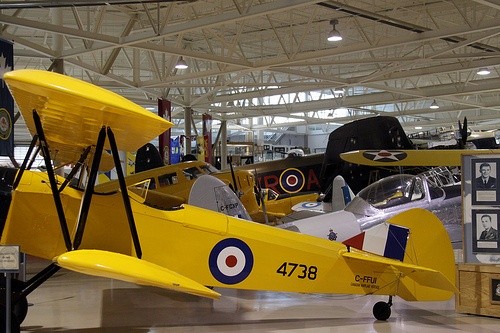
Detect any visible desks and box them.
[455,263,500,318]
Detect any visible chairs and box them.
[387,177,416,207]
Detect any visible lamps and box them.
[175,56,188,69]
[327,19,342,42]
[430,99,439,109]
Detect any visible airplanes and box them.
[0,60,500,333]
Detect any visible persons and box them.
[181,154,218,180]
[479,215,498,240]
[475,164,497,189]
